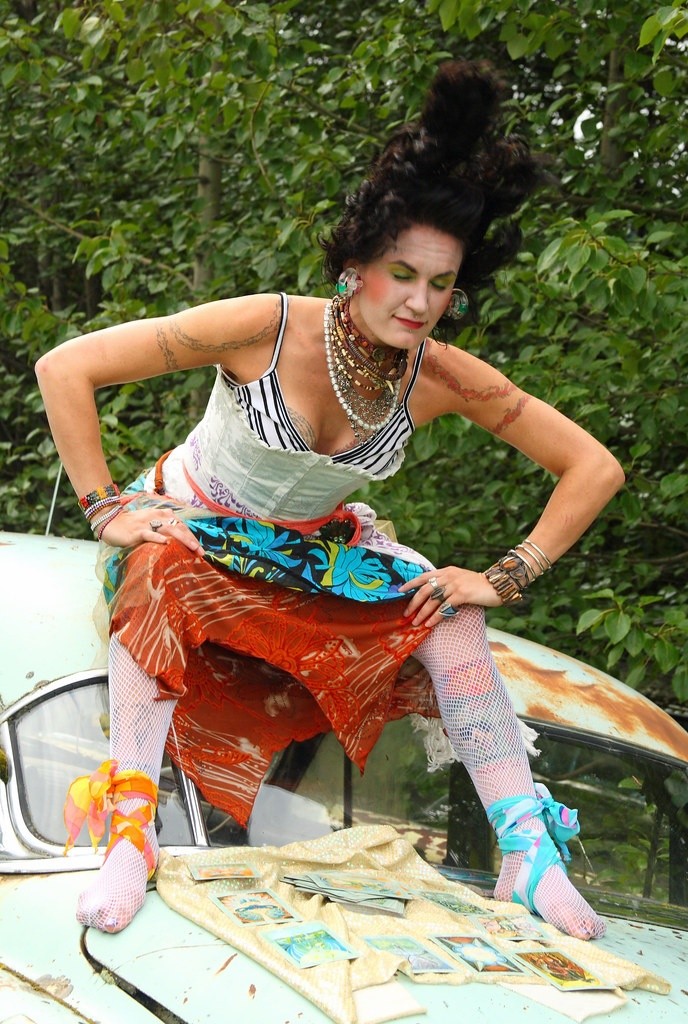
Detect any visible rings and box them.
[439,603,459,618]
[431,586,447,602]
[149,520,162,532]
[169,518,179,526]
[429,577,437,589]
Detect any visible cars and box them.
[1,531,688,1024]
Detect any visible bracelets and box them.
[77,483,124,540]
[485,540,552,607]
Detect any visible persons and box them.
[35,62,626,943]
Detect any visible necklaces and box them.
[324,293,409,446]
